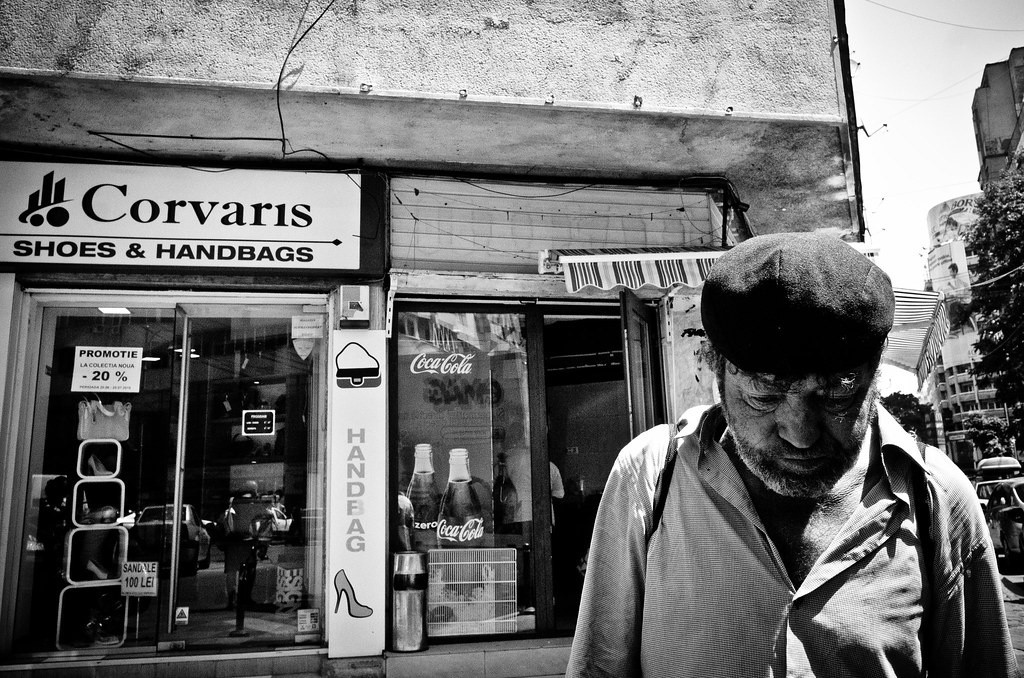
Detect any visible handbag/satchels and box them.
[494,452,518,529]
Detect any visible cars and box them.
[137,503,210,570]
[973,476,1024,553]
[974,457,1022,475]
[224,502,292,539]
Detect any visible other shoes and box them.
[950,328,962,333]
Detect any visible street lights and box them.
[948,263,981,411]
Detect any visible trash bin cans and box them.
[391,550,428,653]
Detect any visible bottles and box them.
[494,451,519,534]
[401,443,484,549]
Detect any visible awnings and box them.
[878,286,949,392]
[543,244,732,293]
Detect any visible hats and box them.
[700,233,895,374]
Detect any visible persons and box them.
[948,263,969,292]
[565,233,1020,678]
[507,446,564,612]
